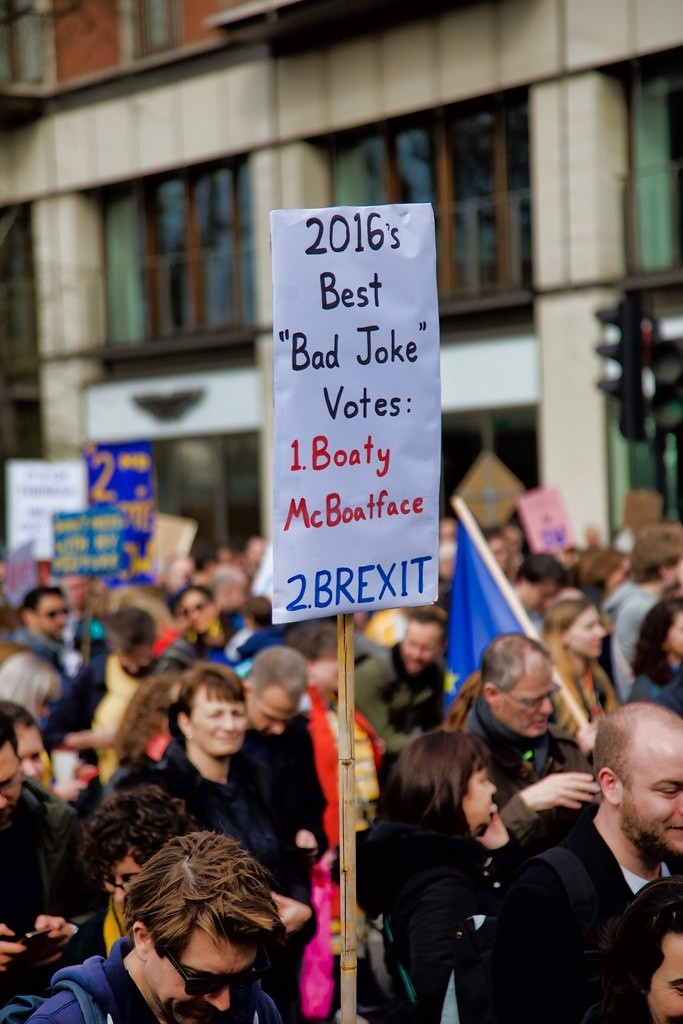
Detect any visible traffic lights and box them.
[594,290,647,444]
[647,336,683,436]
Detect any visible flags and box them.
[441,519,532,716]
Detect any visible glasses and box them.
[495,682,560,709]
[47,607,70,618]
[165,946,271,996]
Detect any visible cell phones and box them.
[21,927,53,946]
[475,812,494,836]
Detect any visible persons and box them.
[0,518,682,1024]
